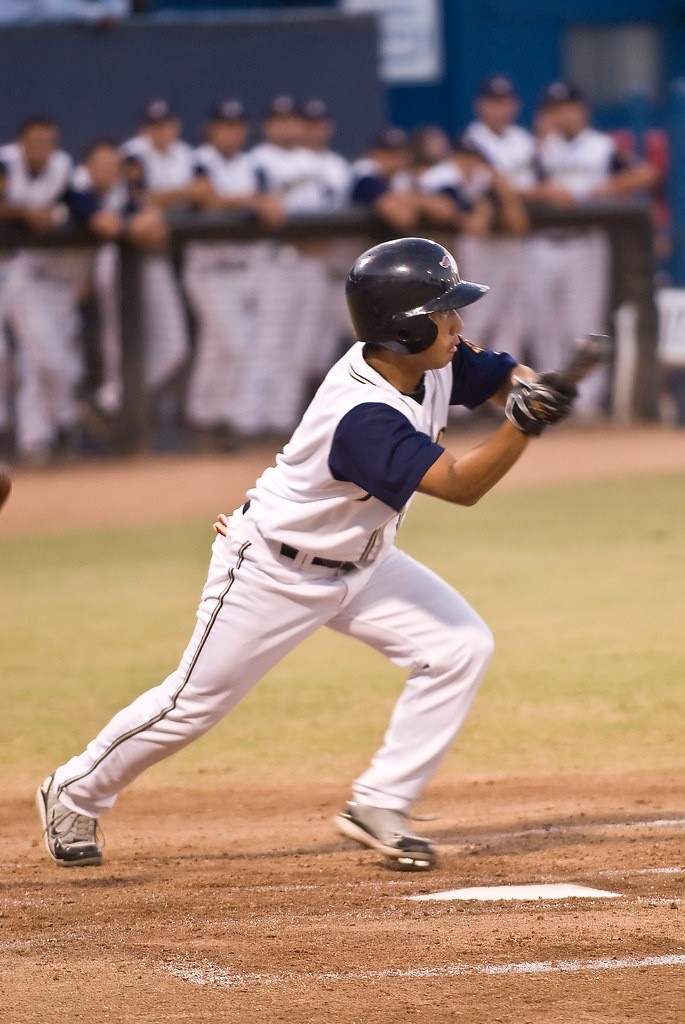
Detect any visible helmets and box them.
[345,237,491,352]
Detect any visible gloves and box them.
[505,371,577,434]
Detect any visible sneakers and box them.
[35,773,106,867]
[335,807,435,867]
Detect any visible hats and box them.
[303,100,328,119]
[475,76,517,99]
[142,99,177,123]
[209,99,246,122]
[371,127,410,151]
[263,95,299,119]
[537,83,581,105]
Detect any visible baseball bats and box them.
[553,331,612,392]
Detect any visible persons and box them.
[35,236,581,869]
[0,79,685,457]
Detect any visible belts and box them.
[243,500,357,571]
